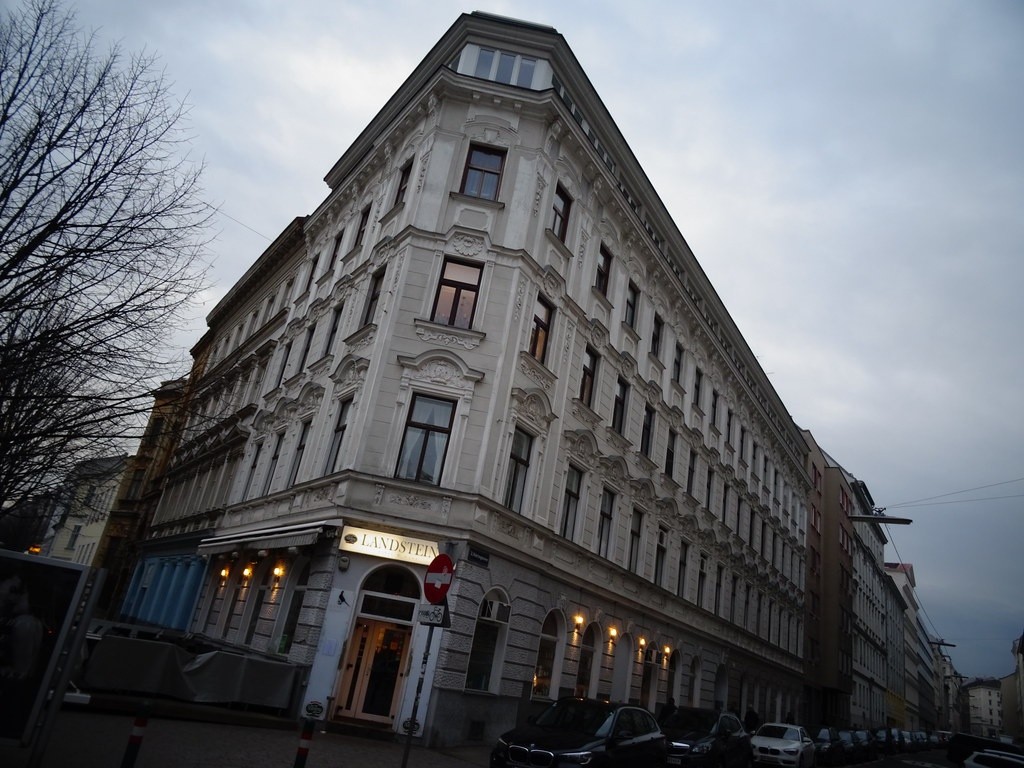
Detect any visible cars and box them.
[947,732,1024,763]
[749,722,817,768]
[806,725,952,768]
[488,694,667,768]
[662,705,751,768]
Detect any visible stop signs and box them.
[423,553,454,604]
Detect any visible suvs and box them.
[959,748,1024,768]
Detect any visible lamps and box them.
[243,568,251,582]
[272,567,281,584]
[664,646,670,658]
[218,569,226,584]
[639,639,646,649]
[610,629,617,640]
[575,616,584,630]
